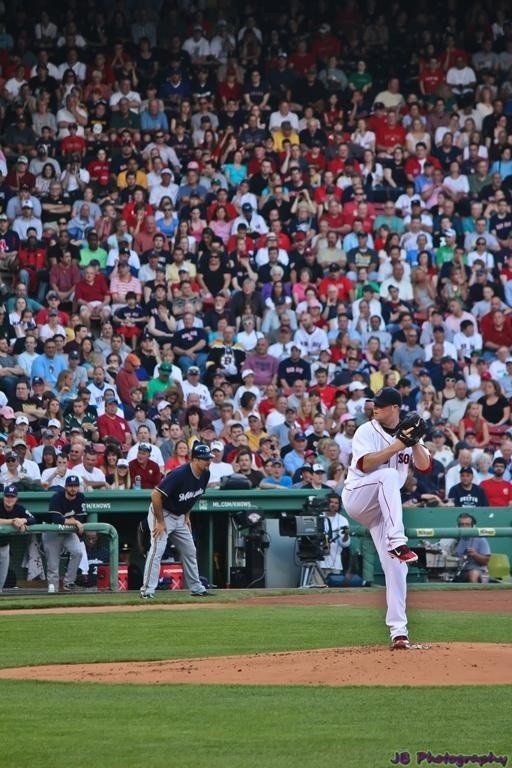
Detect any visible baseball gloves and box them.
[395,411,426,446]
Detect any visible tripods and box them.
[297,562,328,589]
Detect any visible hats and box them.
[112,243,204,287]
[387,281,398,294]
[262,231,283,253]
[355,229,370,240]
[239,195,261,219]
[154,161,184,189]
[65,476,79,486]
[4,486,17,497]
[173,66,253,86]
[190,174,222,207]
[324,259,346,281]
[0,376,409,481]
[3,140,48,236]
[374,98,390,115]
[471,266,490,281]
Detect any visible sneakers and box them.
[191,591,214,596]
[385,545,418,564]
[140,594,156,599]
[390,636,410,648]
[63,583,87,592]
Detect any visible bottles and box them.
[135,474,142,490]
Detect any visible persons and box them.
[340,387,433,650]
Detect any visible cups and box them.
[48,583,56,593]
